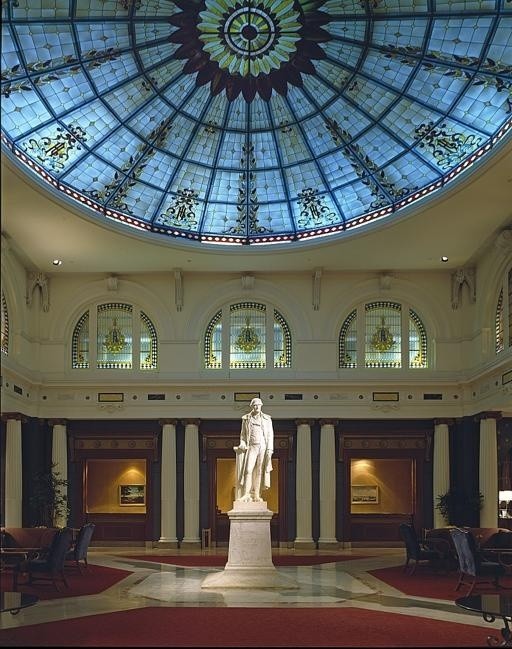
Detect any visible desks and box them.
[456,595,512,648]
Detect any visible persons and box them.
[237,397,274,501]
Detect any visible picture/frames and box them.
[118,484,146,506]
[351,484,378,504]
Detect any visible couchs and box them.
[428,528,512,570]
[0,527,59,568]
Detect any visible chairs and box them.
[13,523,96,592]
[400,523,441,578]
[449,527,504,598]
